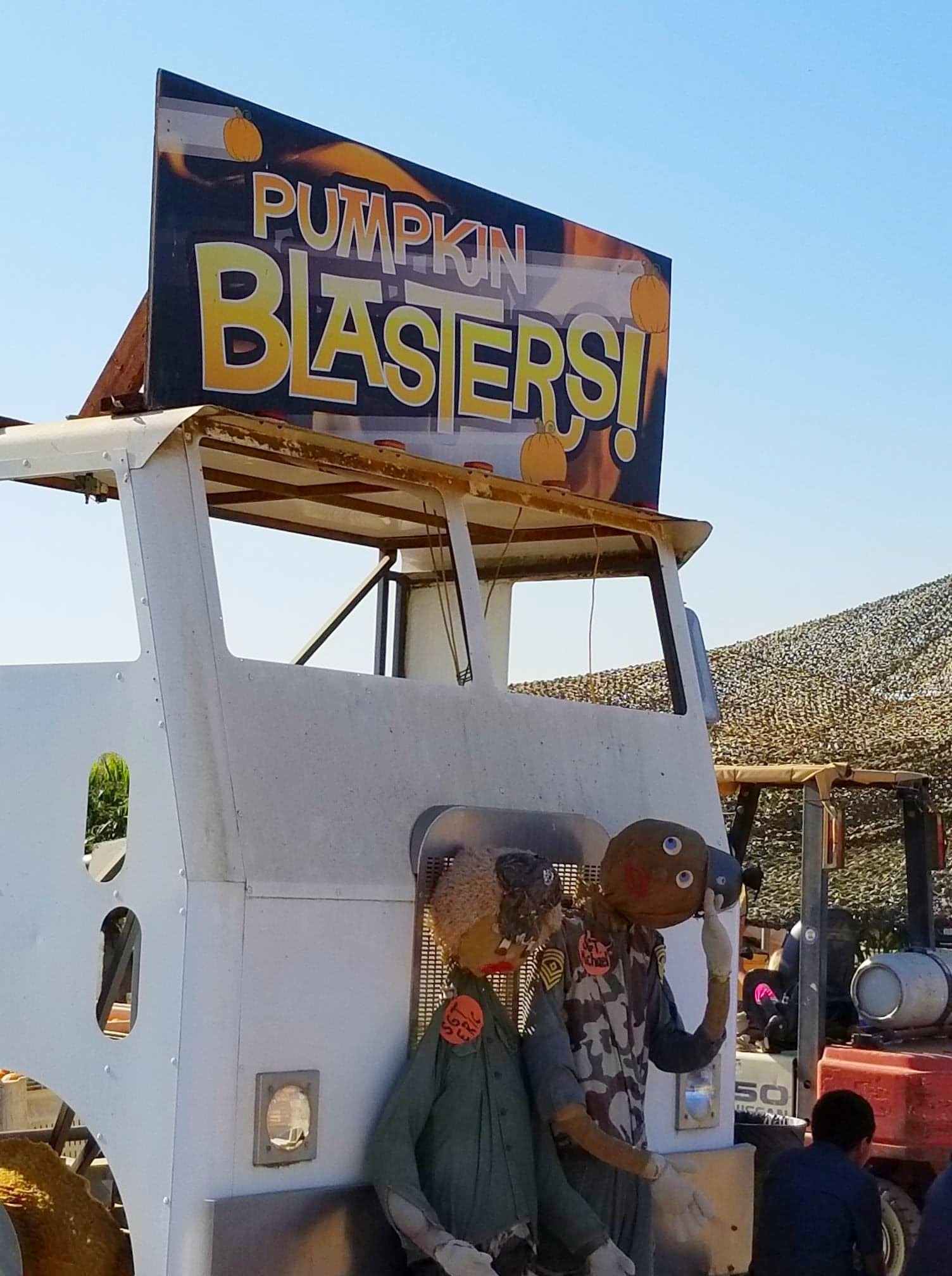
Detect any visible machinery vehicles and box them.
[712,760,951,1275]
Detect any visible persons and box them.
[906,1164,952,1275]
[751,1089,887,1276]
